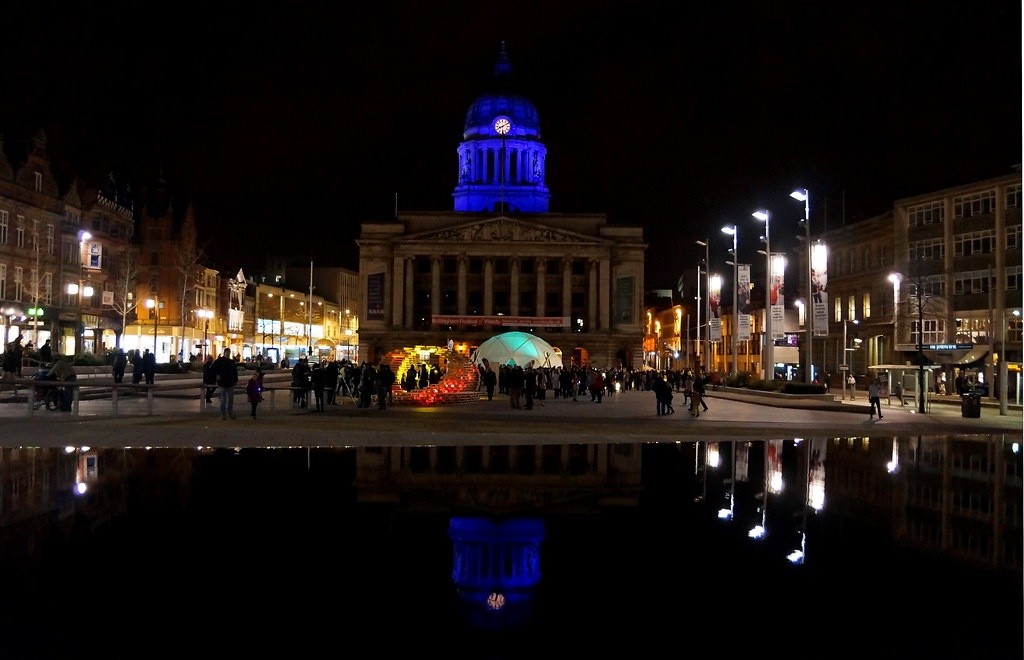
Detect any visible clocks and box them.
[494,116,512,135]
[487,592,505,610]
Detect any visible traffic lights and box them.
[28,305,59,320]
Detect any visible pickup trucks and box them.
[0,358,48,377]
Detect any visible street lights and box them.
[64,229,93,355]
[748,439,770,538]
[887,272,927,415]
[346,330,352,361]
[789,189,812,385]
[199,309,214,361]
[840,319,861,400]
[674,352,679,371]
[696,238,709,374]
[752,209,774,380]
[786,438,812,565]
[722,223,738,380]
[717,441,738,521]
[999,311,1020,415]
[146,276,165,360]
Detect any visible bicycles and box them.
[29,390,62,412]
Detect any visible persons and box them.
[1,334,201,414]
[202,351,292,371]
[813,371,834,392]
[290,355,445,412]
[245,372,263,419]
[709,279,785,319]
[214,347,240,422]
[476,357,731,418]
[936,371,982,400]
[894,381,904,404]
[811,268,825,302]
[203,355,219,404]
[867,379,883,420]
[254,365,266,401]
[847,373,858,400]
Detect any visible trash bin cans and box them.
[961,393,980,418]
